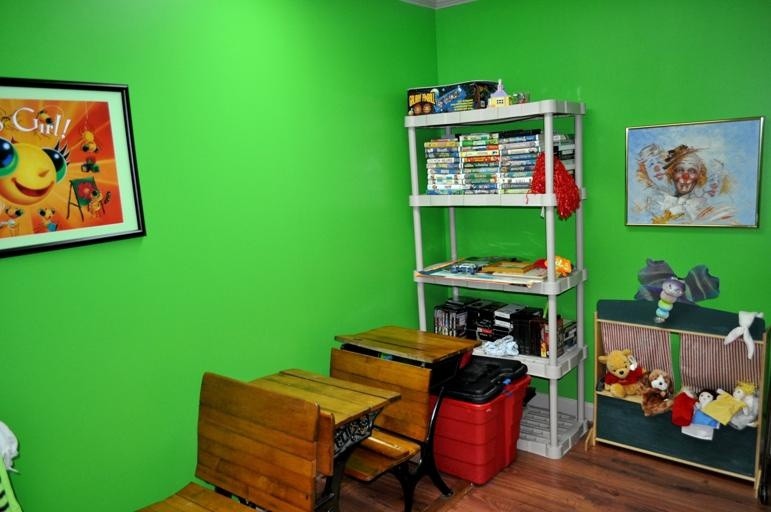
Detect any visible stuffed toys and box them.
[693,389,722,431]
[670,386,698,427]
[598,348,652,399]
[701,380,756,425]
[652,277,685,324]
[635,367,675,418]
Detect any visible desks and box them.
[248,367,403,512]
[335,325,482,368]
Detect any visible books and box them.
[433,296,577,358]
[413,256,546,287]
[414,130,576,196]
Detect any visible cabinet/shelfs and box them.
[591,310,771,503]
[403,100,589,459]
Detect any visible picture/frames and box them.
[0,77,147,258]
[624,115,765,228]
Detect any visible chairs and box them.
[329,348,454,512]
[133,372,335,512]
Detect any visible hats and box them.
[662,145,700,170]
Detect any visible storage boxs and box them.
[429,354,531,486]
